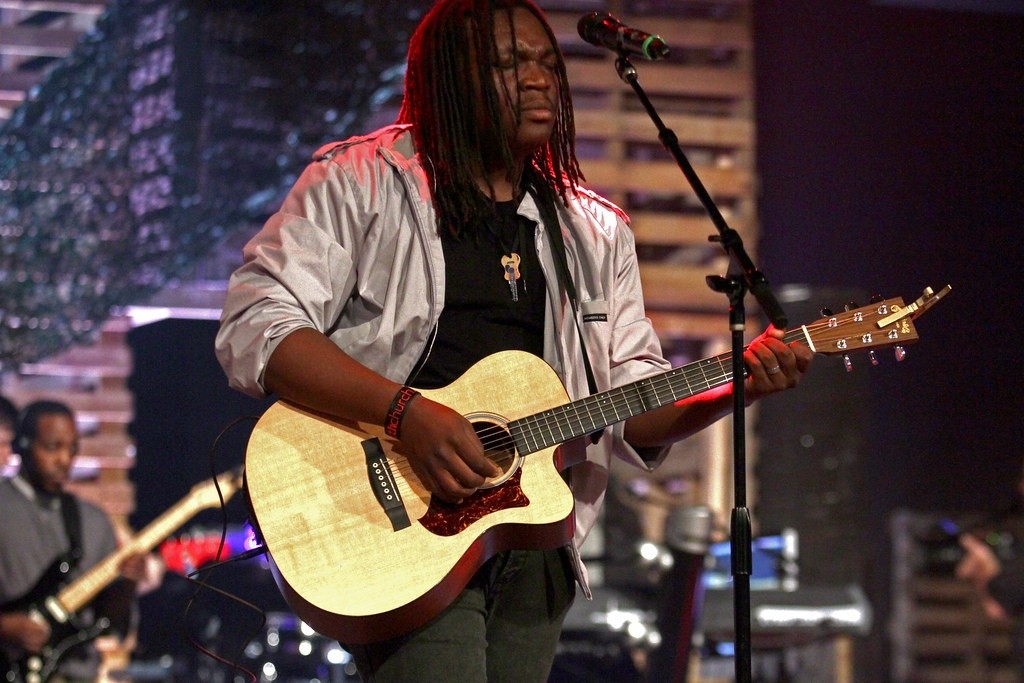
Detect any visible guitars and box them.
[1,460,246,683]
[240,282,956,649]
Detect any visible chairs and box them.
[892,511,1024,683]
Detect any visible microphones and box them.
[578,11,671,60]
[650,503,711,683]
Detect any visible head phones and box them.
[12,401,43,453]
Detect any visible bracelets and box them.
[386,385,420,438]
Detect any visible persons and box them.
[0,399,148,683]
[956,531,1023,620]
[215,0,812,682]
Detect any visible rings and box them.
[767,366,781,375]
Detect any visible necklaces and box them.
[485,200,521,302]
